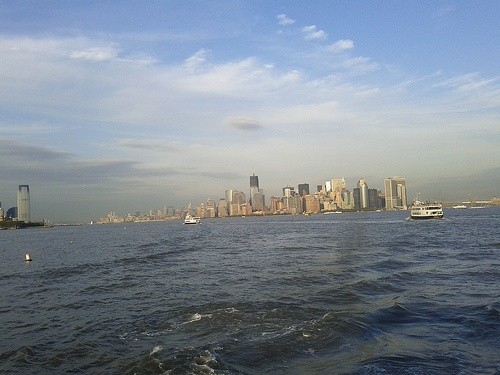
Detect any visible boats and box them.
[470,201,492,210]
[410,205,444,220]
[184,214,201,225]
[323,211,342,215]
[452,202,471,209]
